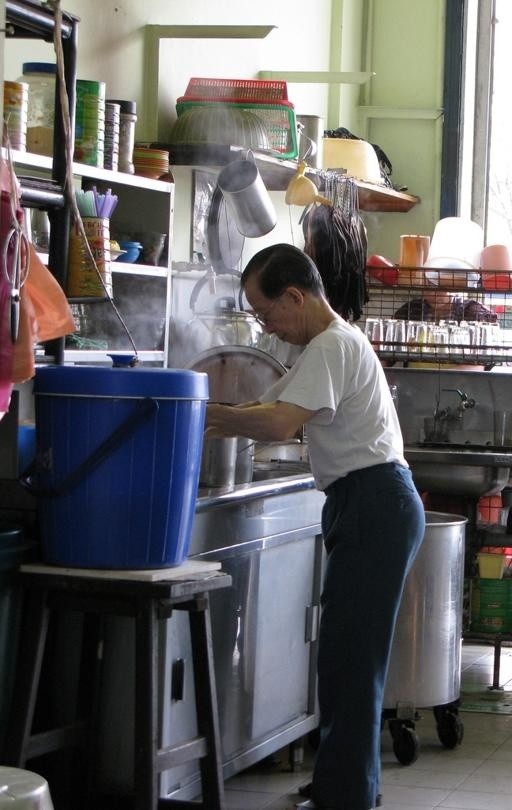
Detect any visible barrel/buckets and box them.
[35,355,209,569]
[423,217,484,286]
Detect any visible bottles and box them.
[35,212,51,248]
[109,98,139,175]
[18,59,67,157]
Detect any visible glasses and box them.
[256,285,291,327]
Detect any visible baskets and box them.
[173,76,299,161]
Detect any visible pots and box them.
[203,426,300,489]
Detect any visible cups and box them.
[216,158,277,237]
[365,317,502,358]
[494,410,512,445]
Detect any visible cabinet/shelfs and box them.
[0,147,175,368]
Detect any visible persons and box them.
[200,241,426,809]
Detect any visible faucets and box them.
[459,399,476,418]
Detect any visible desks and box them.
[12,573,232,810]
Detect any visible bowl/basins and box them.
[120,242,143,263]
[321,137,381,185]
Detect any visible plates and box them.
[110,249,128,260]
[134,145,171,180]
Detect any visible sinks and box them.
[403,446,512,498]
